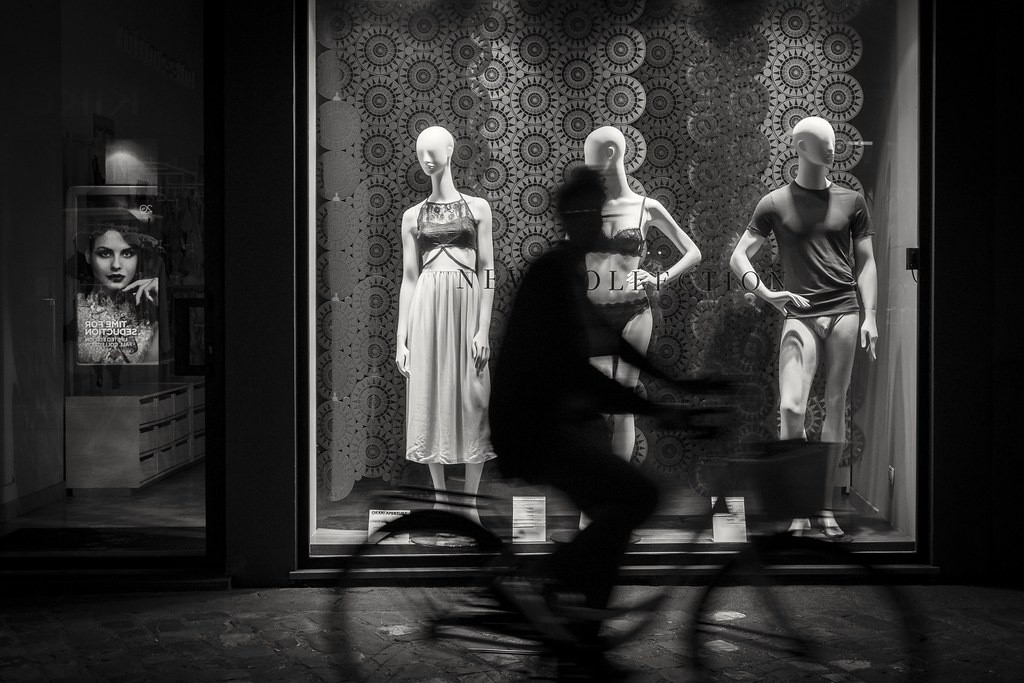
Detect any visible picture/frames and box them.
[63,185,178,396]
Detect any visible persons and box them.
[395,125,498,529]
[730,117,879,540]
[71,215,159,364]
[488,170,746,680]
[578,125,703,529]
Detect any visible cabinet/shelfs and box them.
[125,374,205,468]
[65,382,192,489]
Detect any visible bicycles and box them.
[327,370,935,683]
